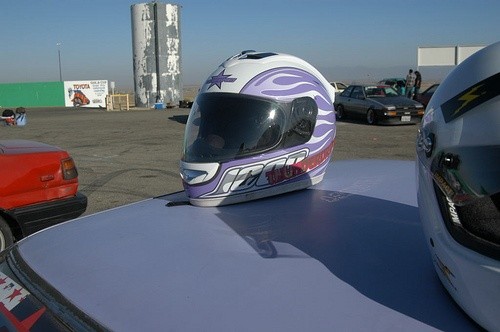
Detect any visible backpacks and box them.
[2,110,15,119]
[16,108,25,113]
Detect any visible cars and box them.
[330,82,347,92]
[415,83,441,108]
[335,83,426,126]
[0,138,88,252]
[0,157,492,332]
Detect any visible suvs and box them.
[375,78,415,95]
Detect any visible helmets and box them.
[416,41,500,332]
[180,50,335,208]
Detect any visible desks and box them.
[106,93,129,111]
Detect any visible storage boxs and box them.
[155,103,163,109]
[0,115,14,126]
[16,113,26,125]
